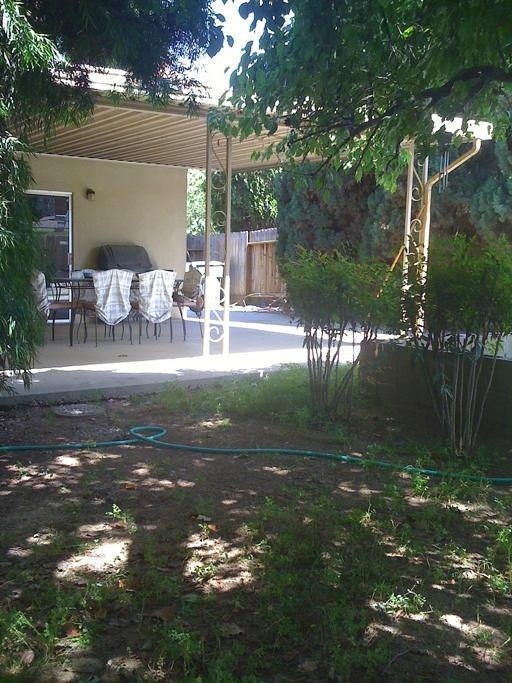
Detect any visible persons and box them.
[30,268,51,322]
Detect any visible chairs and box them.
[43,267,208,348]
